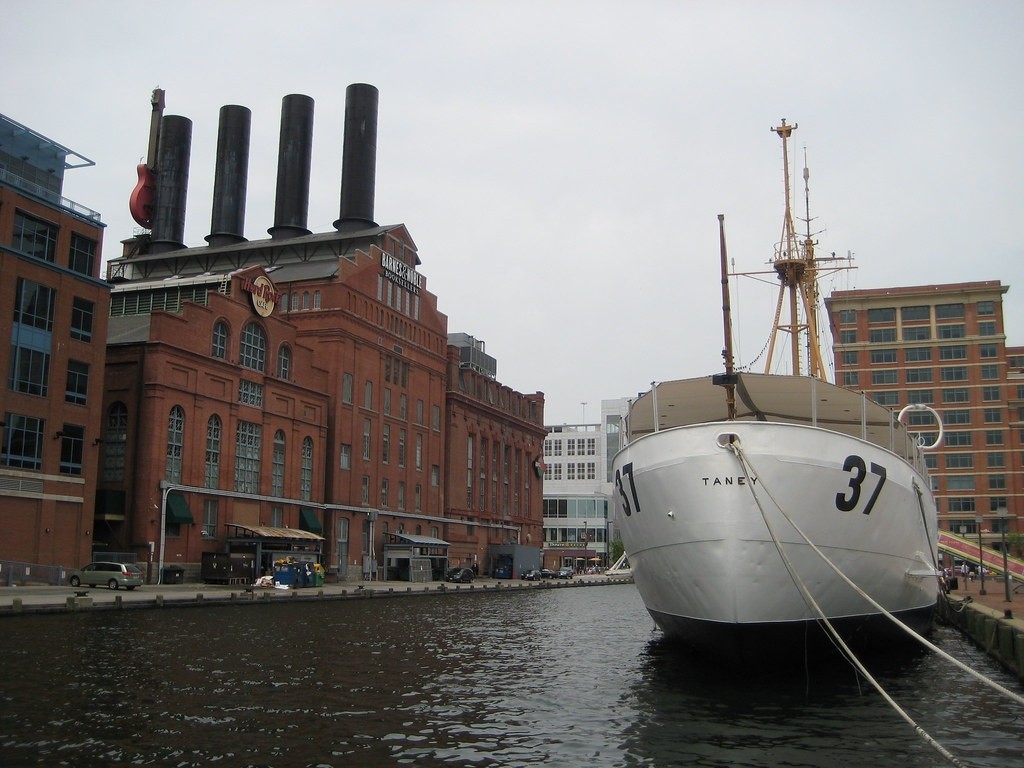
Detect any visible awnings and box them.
[226,523,326,542]
[299,508,322,533]
[383,532,450,549]
[166,492,193,524]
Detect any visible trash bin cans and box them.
[273,560,326,588]
[161,565,185,585]
[950,576,958,590]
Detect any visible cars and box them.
[68,562,143,590]
[521,569,542,581]
[446,567,474,583]
[556,568,573,579]
[945,566,969,576]
[540,570,557,579]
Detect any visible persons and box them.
[576,564,582,574]
[592,565,598,572]
[960,561,975,582]
[939,562,945,577]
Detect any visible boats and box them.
[611,119,940,670]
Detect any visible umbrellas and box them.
[588,558,602,560]
[575,558,585,561]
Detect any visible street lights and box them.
[999,507,1012,602]
[583,520,587,574]
[606,521,613,570]
[960,525,967,590]
[975,515,986,595]
